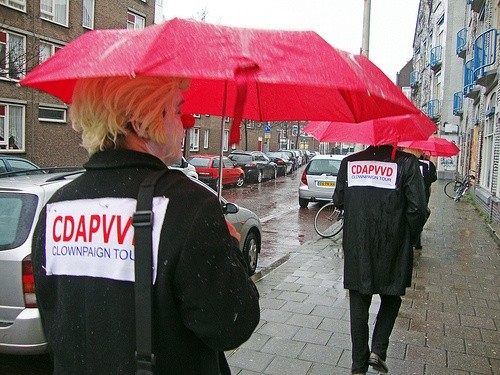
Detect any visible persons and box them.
[30,76,260,375]
[331,143,432,375]
[411,149,438,252]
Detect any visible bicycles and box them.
[443,169,476,203]
[314,200,346,238]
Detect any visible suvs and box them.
[228,149,278,184]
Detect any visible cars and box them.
[341,146,357,155]
[0,165,262,355]
[166,155,198,180]
[265,152,294,176]
[0,154,47,178]
[298,154,350,209]
[185,154,246,192]
[279,148,321,171]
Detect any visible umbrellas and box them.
[14,17,421,209]
[396,137,461,158]
[302,110,439,147]
